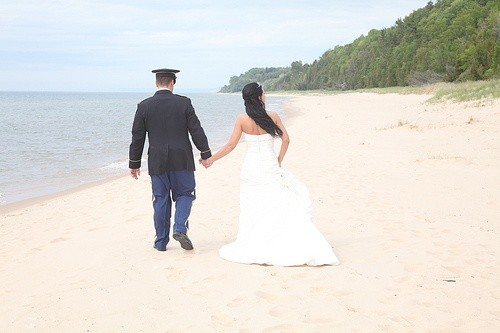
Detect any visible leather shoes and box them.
[173,231,193,251]
[153,244,166,251]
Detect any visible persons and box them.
[128,69,212,252]
[200,82,339,267]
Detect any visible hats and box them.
[151,69,181,79]
